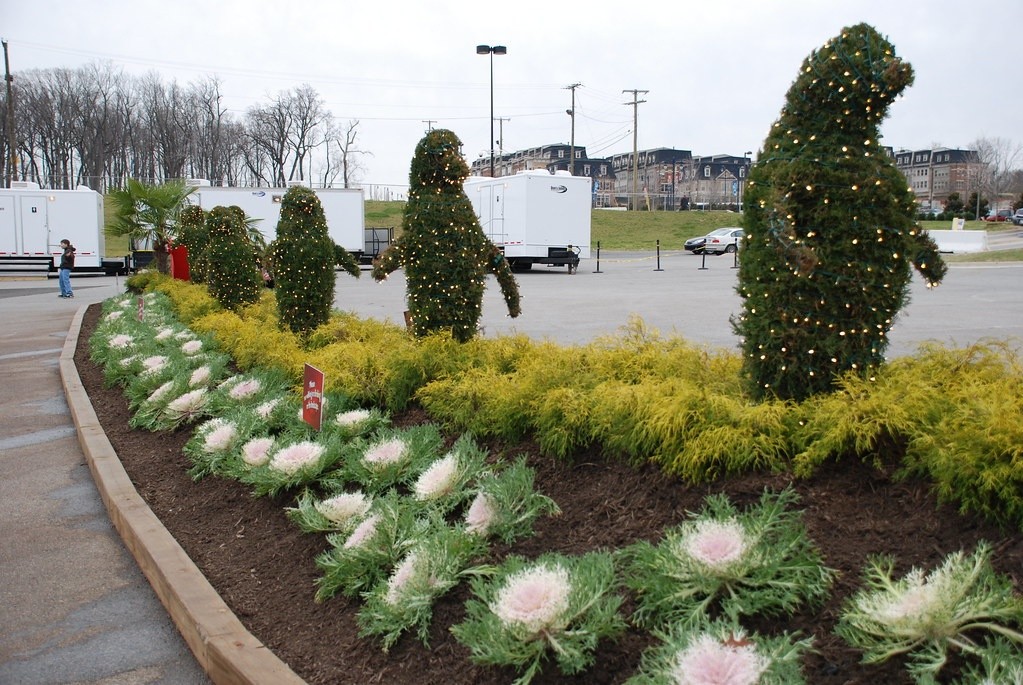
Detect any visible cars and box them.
[1012,208,1023,225]
[704,228,745,253]
[920,199,944,217]
[684,227,727,255]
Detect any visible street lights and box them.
[475,45,508,178]
[744,151,752,189]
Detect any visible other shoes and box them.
[58,295,74,299]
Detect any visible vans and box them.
[987,208,1012,222]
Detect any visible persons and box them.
[58,240,76,298]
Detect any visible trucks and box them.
[129,178,366,255]
[455,167,591,273]
[0,181,106,271]
